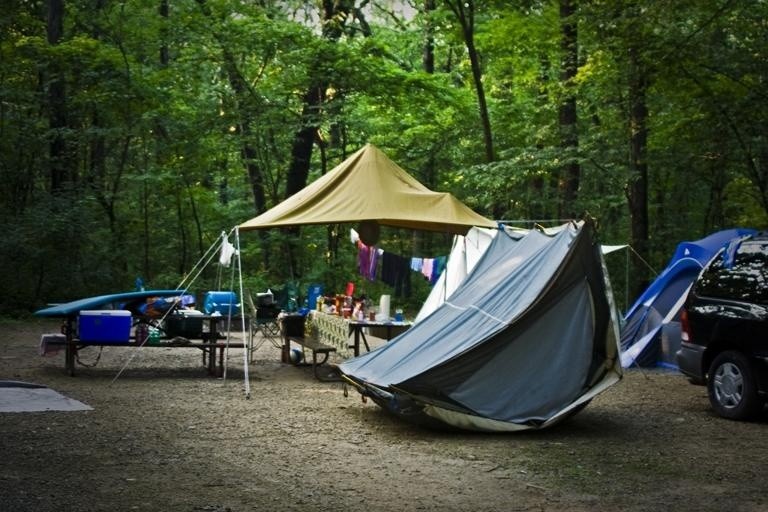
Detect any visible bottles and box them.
[358,310,364,323]
[316,294,324,312]
[369,310,376,321]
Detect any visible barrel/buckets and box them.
[203,290,239,313]
[164,310,203,339]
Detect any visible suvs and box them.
[675,232,768,420]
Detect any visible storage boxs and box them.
[79,310,132,343]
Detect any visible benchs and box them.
[283,333,353,384]
[45,333,247,374]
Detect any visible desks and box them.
[62,312,251,375]
[305,309,414,384]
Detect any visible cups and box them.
[395,309,403,322]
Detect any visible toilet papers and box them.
[380,294,390,318]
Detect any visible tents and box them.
[620,228,763,372]
[332,219,625,438]
